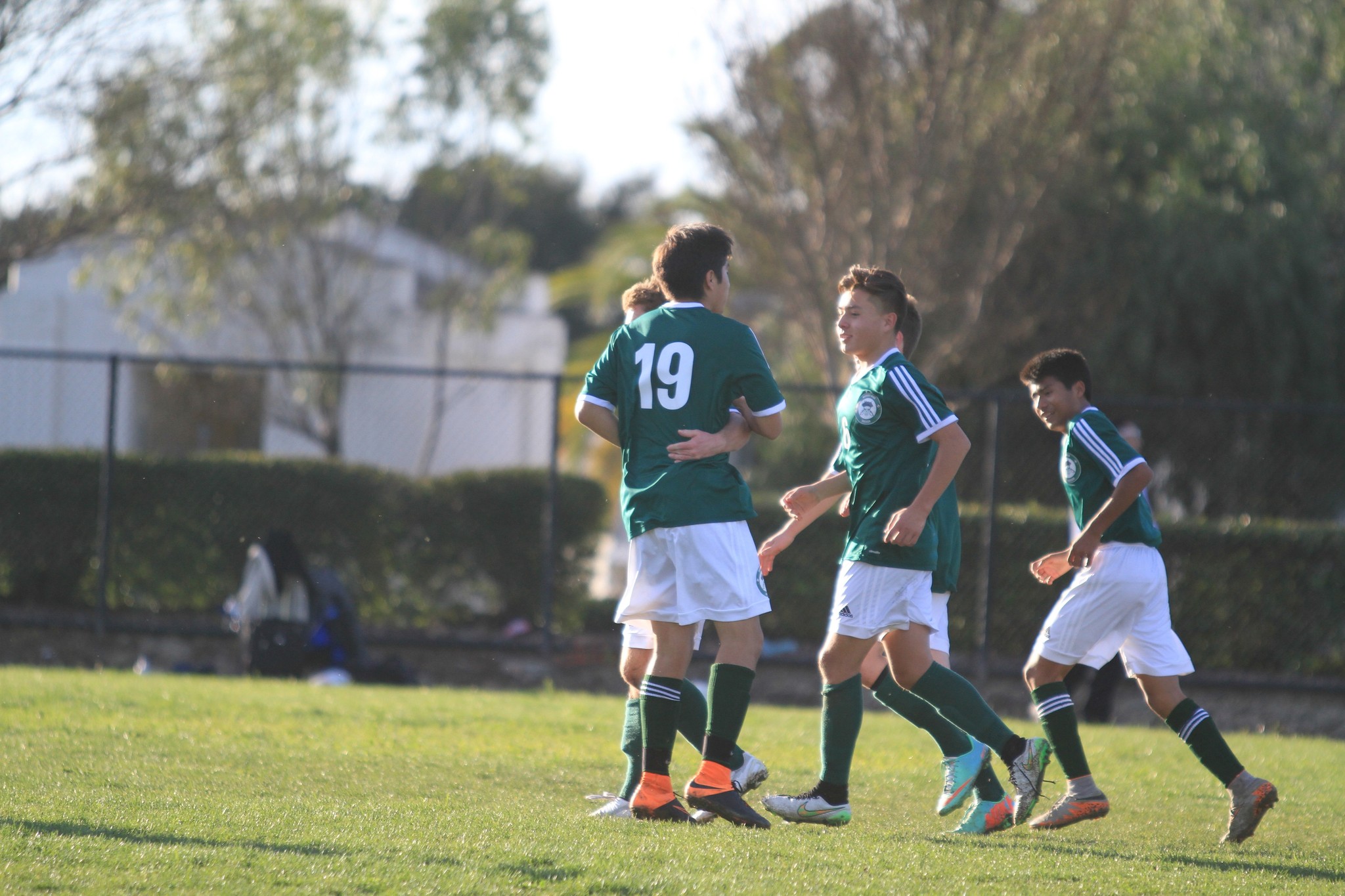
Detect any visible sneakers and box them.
[629,784,697,823]
[685,774,771,830]
[760,787,852,826]
[585,792,632,818]
[948,792,1016,833]
[1030,789,1110,829]
[691,752,770,824]
[937,734,992,817]
[1007,737,1054,826]
[1219,777,1279,846]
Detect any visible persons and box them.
[762,263,1050,826]
[756,296,1015,834]
[586,277,769,825]
[1021,350,1279,846]
[574,223,785,829]
